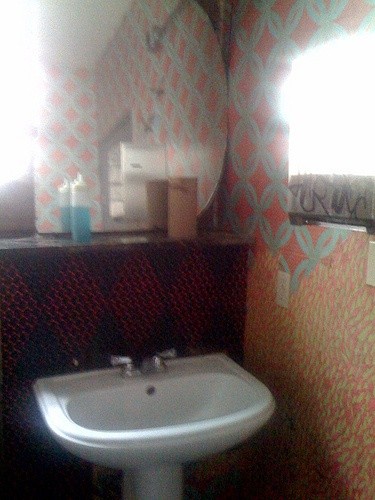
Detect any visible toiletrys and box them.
[59,175,72,235]
[147,179,169,234]
[168,175,197,239]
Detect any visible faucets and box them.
[141,350,167,373]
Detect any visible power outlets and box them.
[275,269,290,309]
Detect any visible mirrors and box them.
[286,27,375,235]
[36,0,226,235]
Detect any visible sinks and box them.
[66,372,259,431]
